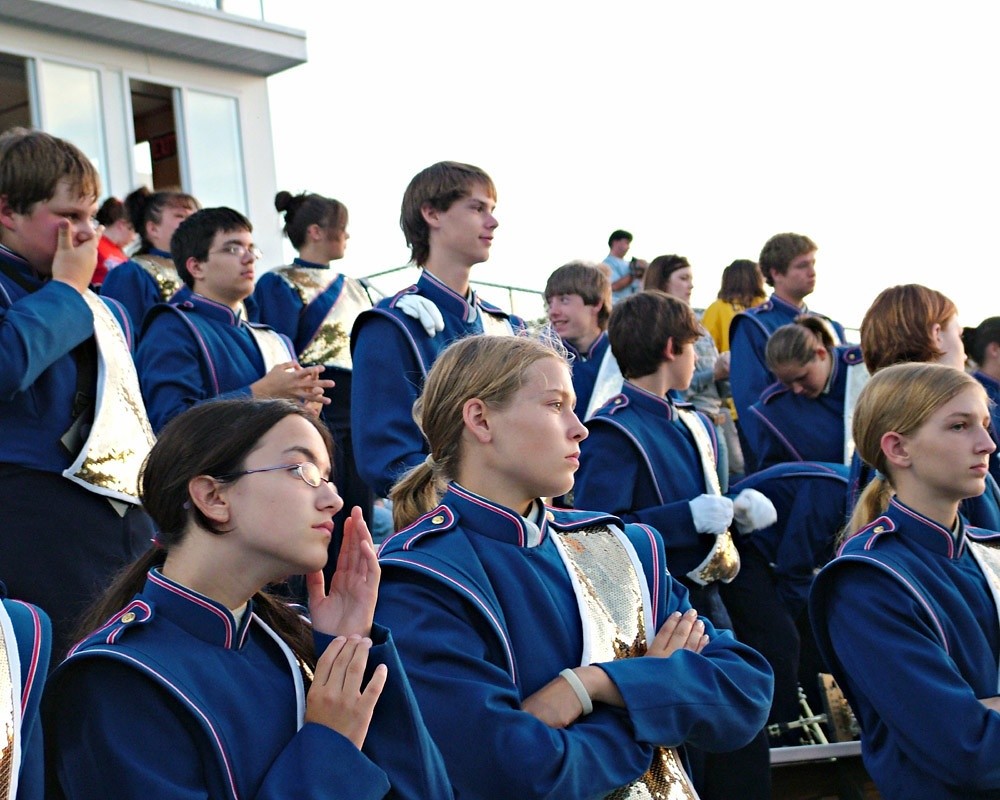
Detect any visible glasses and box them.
[209,245,264,260]
[183,462,337,508]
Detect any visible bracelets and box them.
[560,668,593,715]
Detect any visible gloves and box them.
[689,494,733,536]
[732,488,778,533]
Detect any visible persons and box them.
[0,124,1000,800]
